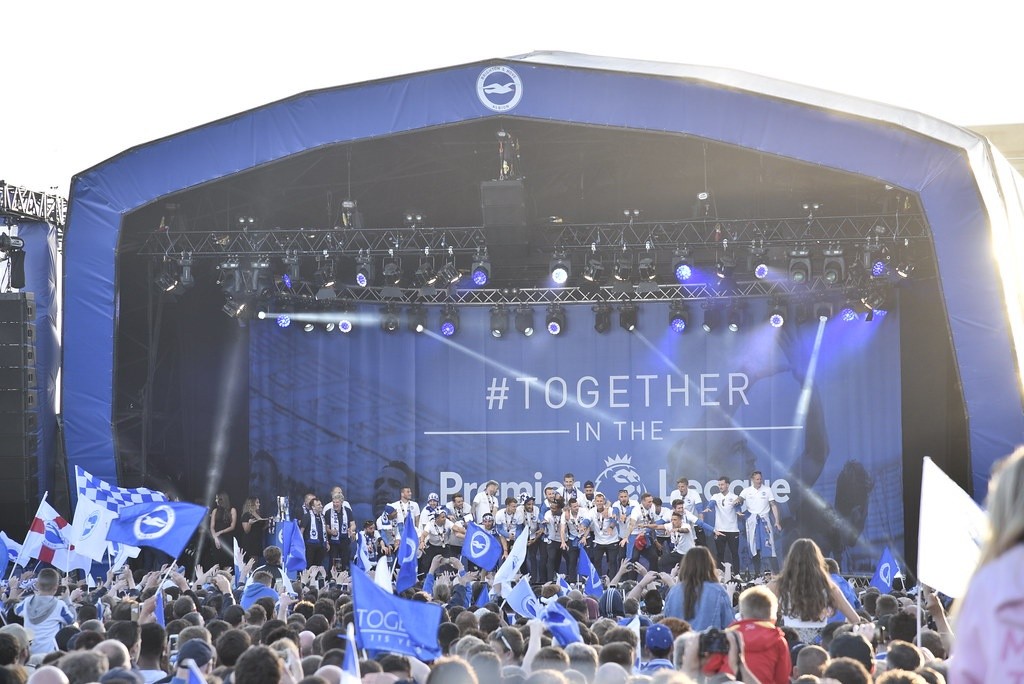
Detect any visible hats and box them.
[177,638,214,669]
[644,624,674,649]
[598,588,626,618]
[0,623,33,651]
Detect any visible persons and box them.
[0,444,1023,684]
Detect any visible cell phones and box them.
[170,635,178,654]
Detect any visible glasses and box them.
[495,627,513,653]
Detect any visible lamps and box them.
[638,258,657,282]
[717,256,735,278]
[549,260,572,284]
[753,240,914,328]
[545,312,566,336]
[514,313,535,337]
[583,258,605,283]
[439,314,459,336]
[613,259,633,282]
[220,258,376,336]
[408,315,427,334]
[438,262,462,288]
[594,312,612,333]
[726,307,744,333]
[471,262,491,286]
[383,313,402,333]
[620,310,639,331]
[671,256,695,282]
[702,310,720,333]
[667,309,688,332]
[154,260,194,292]
[416,263,438,286]
[383,263,403,285]
[489,314,510,338]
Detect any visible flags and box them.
[505,575,539,620]
[233,536,246,587]
[351,564,442,663]
[76,464,170,514]
[69,494,118,564]
[492,527,531,585]
[106,501,210,560]
[576,546,604,600]
[396,511,419,594]
[275,521,283,552]
[461,521,502,573]
[286,520,307,571]
[283,519,294,565]
[540,595,581,649]
[475,585,491,608]
[916,455,991,599]
[18,501,93,578]
[869,547,899,594]
[0,529,31,569]
[340,622,362,679]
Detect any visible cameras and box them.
[628,565,637,570]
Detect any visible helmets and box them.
[434,510,447,517]
[516,492,536,504]
[426,492,440,502]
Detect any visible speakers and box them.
[481,178,535,288]
[0,291,41,543]
